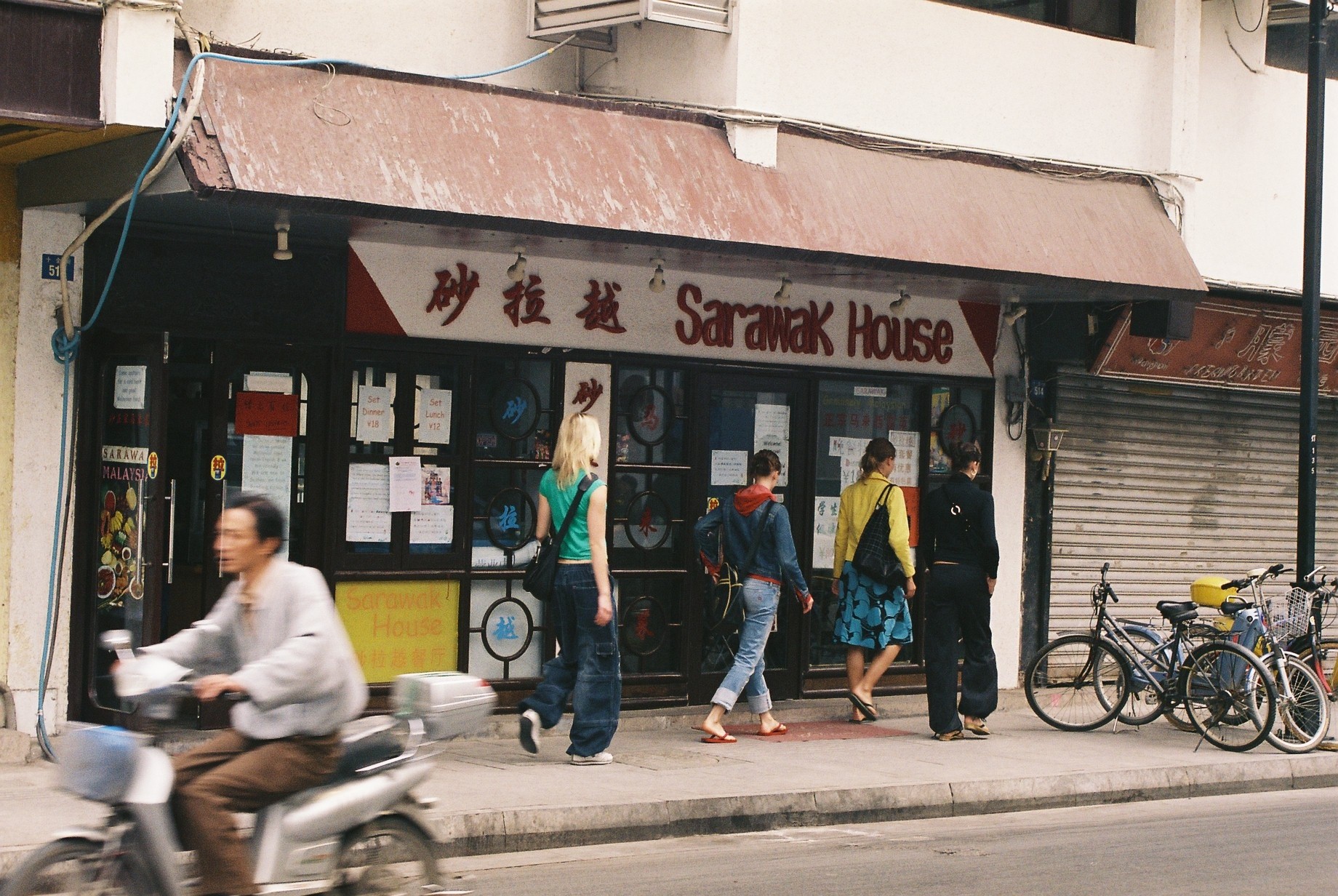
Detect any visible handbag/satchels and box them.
[703,563,746,636]
[522,535,560,601]
[851,484,907,588]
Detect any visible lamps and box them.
[1028,415,1070,484]
[274,224,293,261]
[507,244,529,283]
[647,258,667,295]
[889,284,912,317]
[1002,296,1028,326]
[774,271,793,305]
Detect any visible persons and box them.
[517,414,622,767]
[831,438,916,725]
[916,442,999,742]
[686,448,814,743]
[112,490,370,896]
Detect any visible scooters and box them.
[1092,561,1338,752]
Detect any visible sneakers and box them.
[570,752,613,765]
[519,708,541,754]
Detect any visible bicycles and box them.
[1024,563,1281,753]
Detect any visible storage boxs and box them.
[1189,577,1238,610]
[387,670,498,742]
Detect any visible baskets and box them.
[1261,587,1313,639]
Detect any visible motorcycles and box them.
[0,629,501,895]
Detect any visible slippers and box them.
[963,723,990,736]
[939,731,964,741]
[847,691,878,721]
[701,732,737,743]
[758,723,788,737]
[849,717,873,724]
